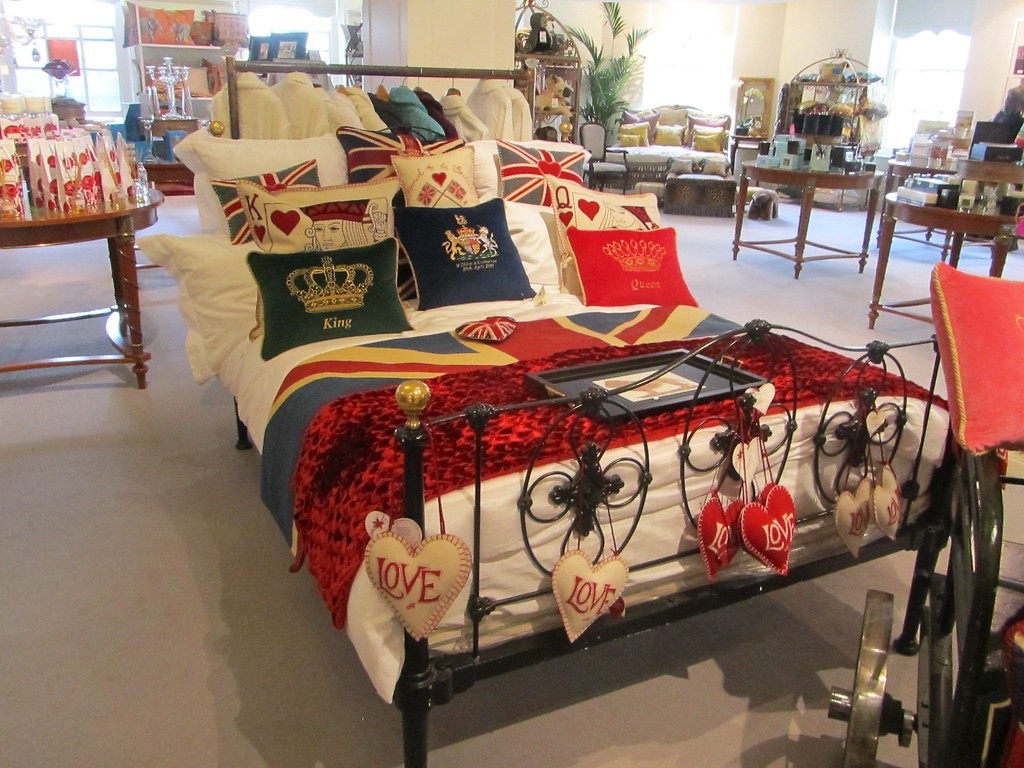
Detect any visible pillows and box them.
[123,3,251,51]
[930,261,1024,457]
[139,129,700,384]
[699,156,727,177]
[617,109,724,153]
[665,157,693,174]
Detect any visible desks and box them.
[731,160,884,278]
[868,152,1024,329]
[730,131,764,177]
[1,184,164,389]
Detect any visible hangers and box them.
[242,56,516,101]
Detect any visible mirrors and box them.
[736,76,776,138]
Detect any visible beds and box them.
[139,116,960,768]
[605,105,730,191]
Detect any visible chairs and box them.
[579,121,630,193]
